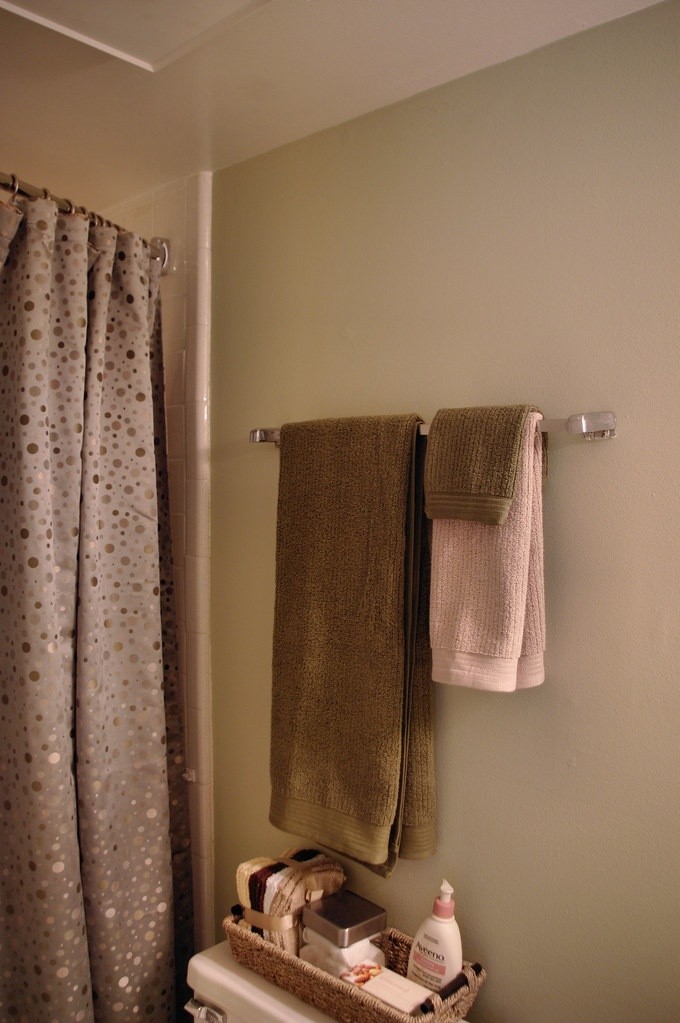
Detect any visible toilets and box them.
[183,939,334,1023]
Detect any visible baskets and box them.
[223,911,486,1023]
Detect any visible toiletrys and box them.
[406,879,463,992]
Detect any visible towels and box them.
[235,845,347,958]
[268,413,439,878]
[423,405,551,695]
